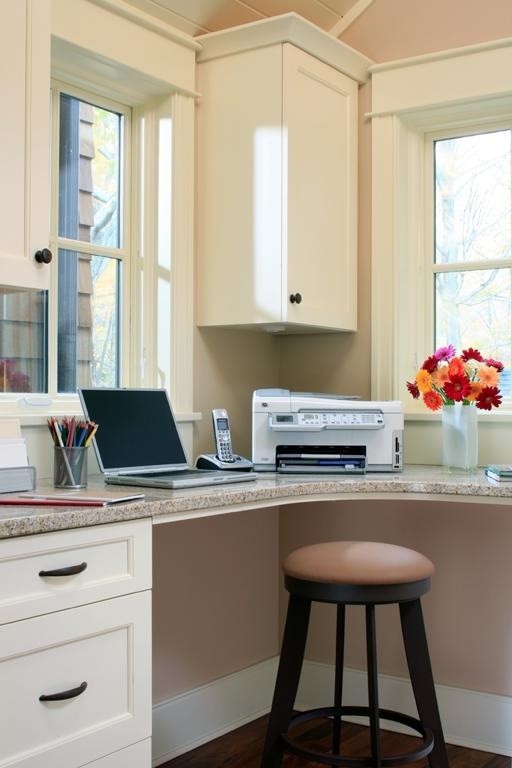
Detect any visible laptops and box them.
[75,386,258,488]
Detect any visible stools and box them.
[259,539,452,768]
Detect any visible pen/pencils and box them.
[47,416,99,488]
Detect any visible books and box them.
[485,464,512,482]
[0,488,145,506]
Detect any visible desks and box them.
[2,463,510,768]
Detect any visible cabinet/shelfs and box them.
[0,1,58,297]
[197,41,361,339]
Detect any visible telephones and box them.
[194,408,253,472]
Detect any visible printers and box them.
[250,388,405,474]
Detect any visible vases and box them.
[438,405,481,476]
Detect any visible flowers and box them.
[406,340,505,417]
